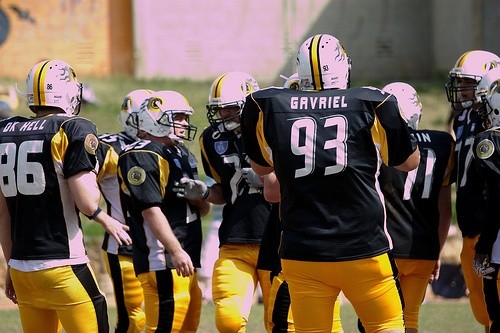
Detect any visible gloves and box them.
[171,177,209,201]
[472,253,495,280]
[241,168,264,189]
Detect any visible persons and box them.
[0,59,133,333]
[445,49,500,333]
[241,34,422,333]
[97,71,455,333]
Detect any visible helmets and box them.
[380,82,423,130]
[15,59,83,115]
[445,50,500,111]
[117,88,198,143]
[206,72,260,136]
[279,34,351,90]
